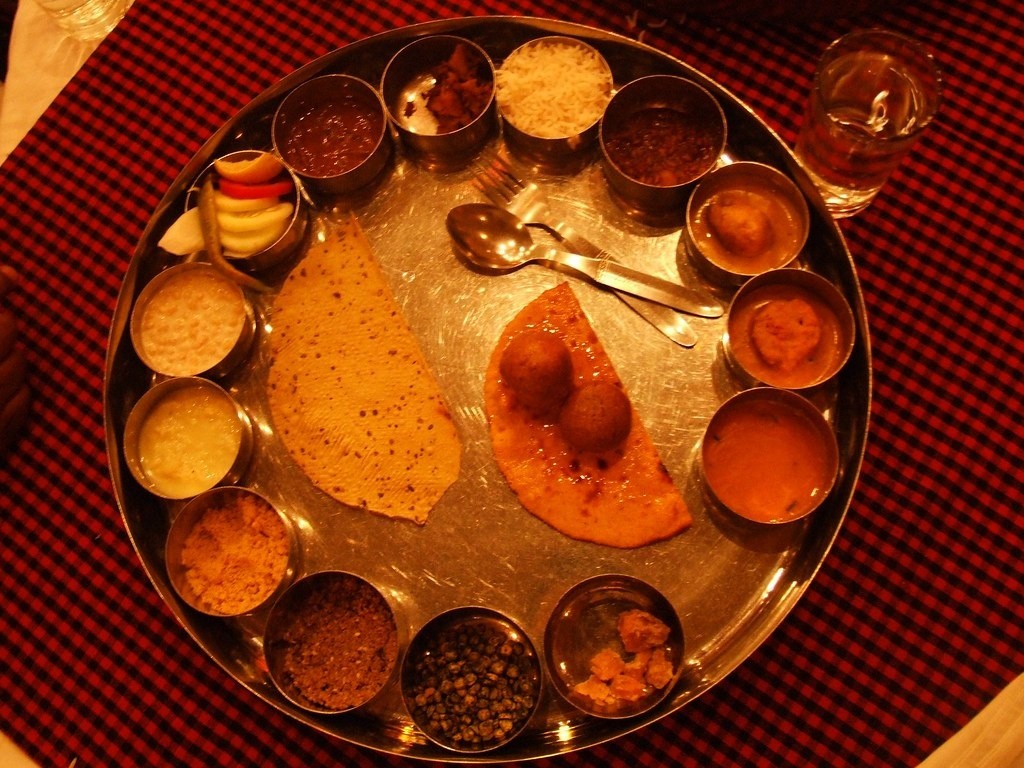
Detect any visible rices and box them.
[494,40,611,140]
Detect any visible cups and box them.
[793,31,943,219]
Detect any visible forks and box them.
[467,146,699,347]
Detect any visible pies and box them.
[266,210,462,526]
[485,282,694,549]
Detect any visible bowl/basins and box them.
[271,74,390,198]
[599,75,726,204]
[381,35,495,160]
[695,387,839,527]
[399,606,541,752]
[544,574,684,719]
[724,269,854,387]
[262,571,410,713]
[185,152,307,274]
[130,259,257,381]
[123,375,254,500]
[166,486,300,618]
[684,162,810,284]
[495,39,613,158]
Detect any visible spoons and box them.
[445,203,725,318]
